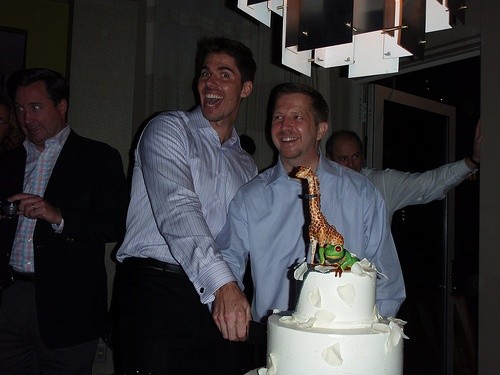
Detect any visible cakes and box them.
[258,253,410,375]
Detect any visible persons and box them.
[209,82,409,374]
[0,67,130,375]
[110,35,260,375]
[325,117,483,218]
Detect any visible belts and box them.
[135,258,188,275]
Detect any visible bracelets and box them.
[468,156,480,167]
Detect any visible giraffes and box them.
[296,165,344,264]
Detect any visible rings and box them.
[31,203,36,210]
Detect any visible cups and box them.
[0,202,18,224]
